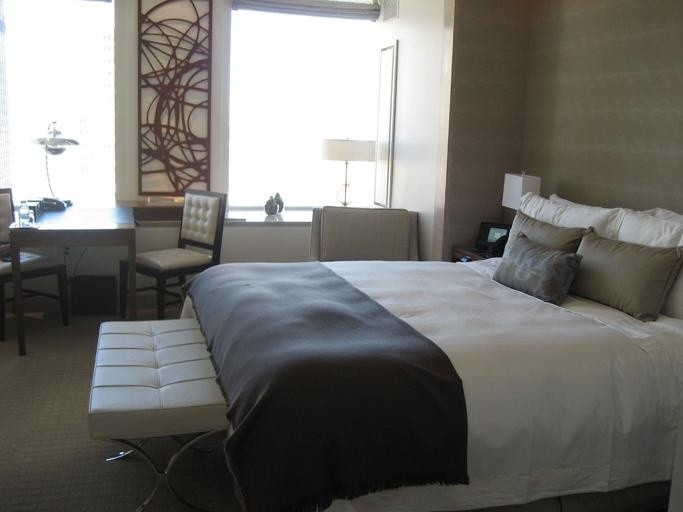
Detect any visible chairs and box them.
[0,190,69,340]
[118,189,226,318]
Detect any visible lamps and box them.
[36,122,79,210]
[501,169,541,210]
[322,138,374,206]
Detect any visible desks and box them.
[9,208,135,356]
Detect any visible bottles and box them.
[18,200,30,228]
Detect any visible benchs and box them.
[90,318,229,511]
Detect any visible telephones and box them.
[41,197,66,210]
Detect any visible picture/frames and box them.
[476,222,510,249]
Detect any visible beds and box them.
[181,257,683,512]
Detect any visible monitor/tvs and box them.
[486,225,507,242]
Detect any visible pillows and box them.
[618,212,683,249]
[503,212,579,261]
[520,193,623,242]
[574,227,680,323]
[491,232,581,305]
